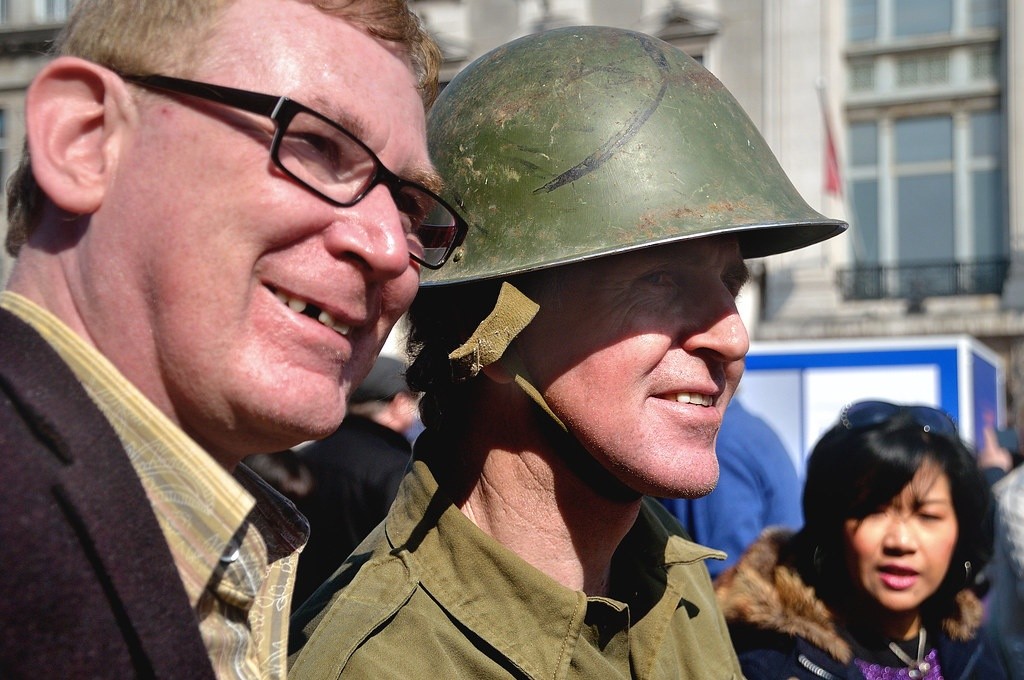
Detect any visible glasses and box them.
[840,398,958,441]
[111,69,468,272]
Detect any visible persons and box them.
[307,342,429,602]
[285,23,855,680]
[0,0,472,680]
[651,396,1024,680]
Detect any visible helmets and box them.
[416,23,849,288]
